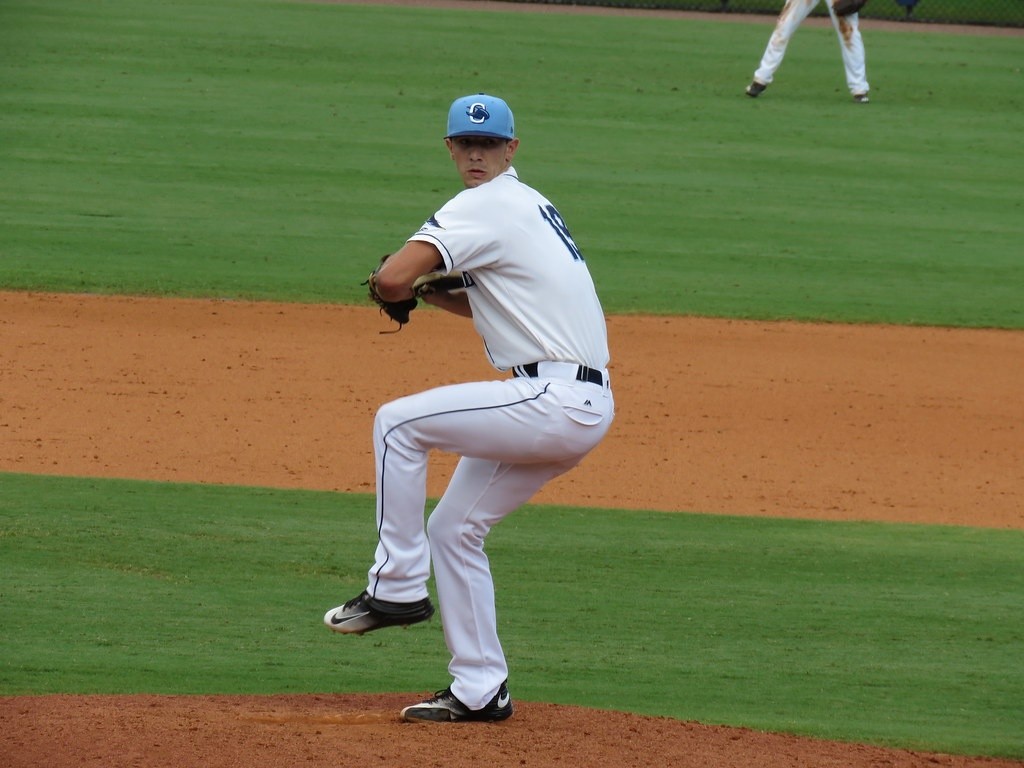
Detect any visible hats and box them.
[444,93,515,140]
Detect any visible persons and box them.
[324,93,614,720]
[745,0,871,103]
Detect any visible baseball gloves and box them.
[365,254,418,325]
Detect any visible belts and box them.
[512,362,609,387]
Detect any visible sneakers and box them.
[323,589,436,634]
[400,678,514,723]
[746,80,765,96]
[854,94,869,104]
